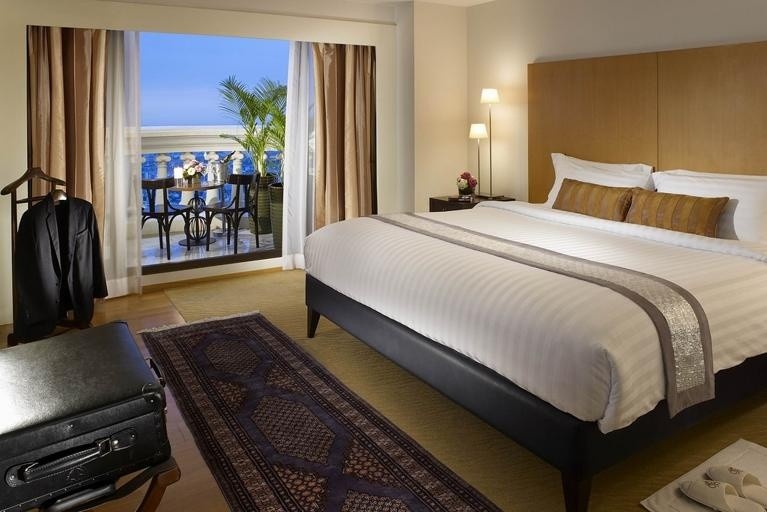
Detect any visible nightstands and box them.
[428,191,516,212]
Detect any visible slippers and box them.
[706,465,767,509]
[680,480,767,512]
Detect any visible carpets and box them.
[639,438,767,511]
[137,308,505,512]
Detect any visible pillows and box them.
[545,152,767,245]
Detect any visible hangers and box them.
[51,185,69,202]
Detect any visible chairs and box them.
[141,176,193,260]
[201,172,260,254]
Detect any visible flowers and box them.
[456,170,478,191]
[182,159,205,180]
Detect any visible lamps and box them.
[469,86,502,199]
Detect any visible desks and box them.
[167,180,226,246]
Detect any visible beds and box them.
[302,201,767,512]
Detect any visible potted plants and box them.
[216,72,287,251]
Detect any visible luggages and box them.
[0,320,171,512]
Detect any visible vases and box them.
[459,188,474,197]
[188,174,201,184]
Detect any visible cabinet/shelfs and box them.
[38,453,183,512]
